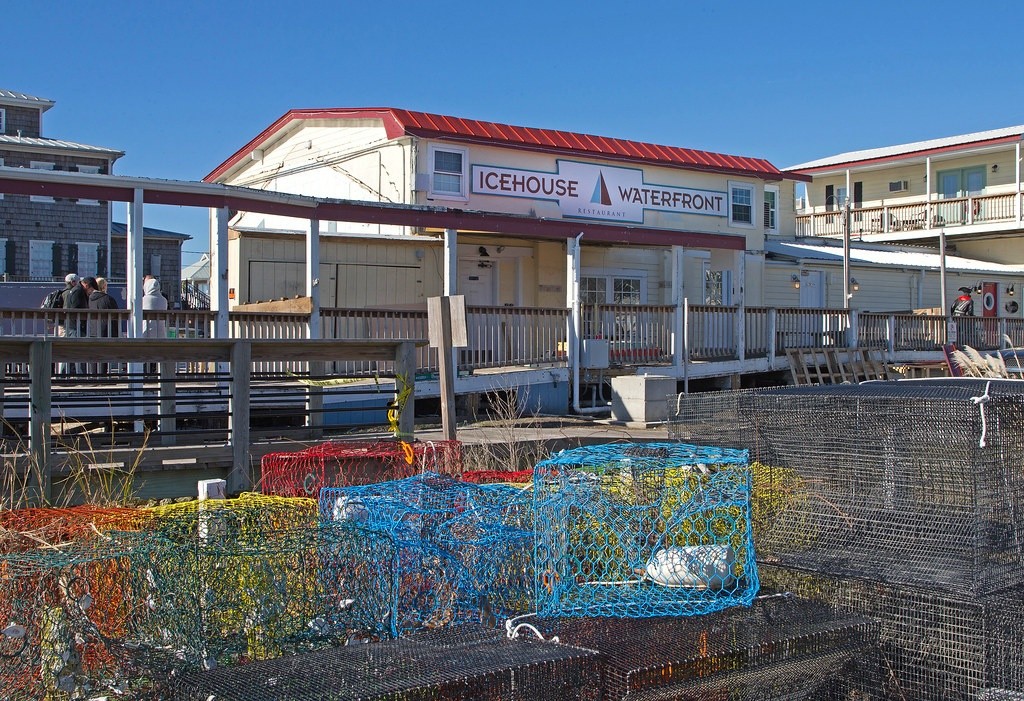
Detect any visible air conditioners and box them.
[764,201,771,229]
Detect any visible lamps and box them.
[974,280,982,294]
[1006,280,1015,296]
[851,278,859,291]
[992,165,997,172]
[923,174,927,182]
[792,275,800,289]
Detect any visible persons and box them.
[59,277,99,376]
[88,277,119,376]
[53,273,79,373]
[141,279,167,342]
[143,275,170,313]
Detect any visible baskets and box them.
[0,382,1024,699]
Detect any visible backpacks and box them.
[41,289,70,309]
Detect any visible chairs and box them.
[879,207,933,230]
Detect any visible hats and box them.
[84,276,99,291]
[64,273,84,283]
